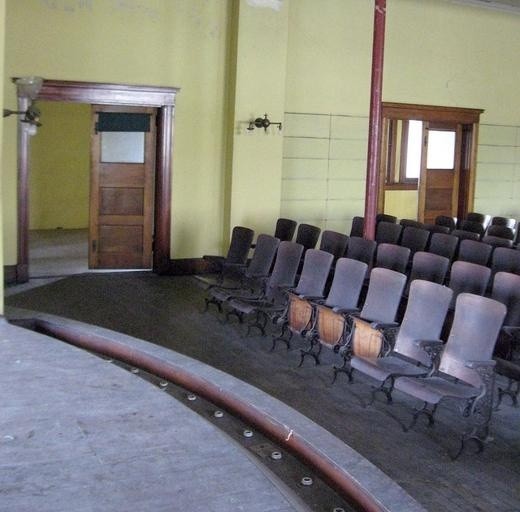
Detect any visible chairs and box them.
[197,212,519,461]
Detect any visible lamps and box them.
[1,74,42,137]
[246,116,283,136]
